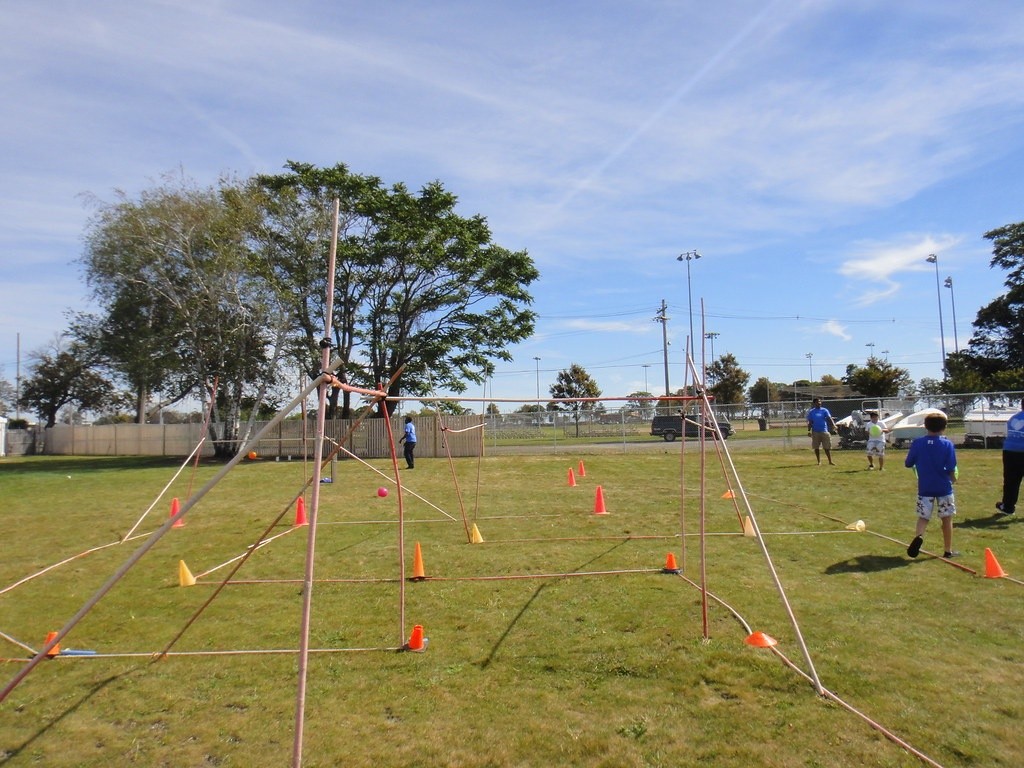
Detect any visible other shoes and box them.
[907,535,923,558]
[943,550,961,558]
[996,502,1016,516]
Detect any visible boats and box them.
[962,405,1023,439]
[835,408,904,441]
[892,408,948,440]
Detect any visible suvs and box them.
[649,415,735,442]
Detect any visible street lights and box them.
[641,364,651,393]
[805,352,814,382]
[676,248,702,450]
[881,351,890,359]
[865,343,875,357]
[704,332,720,387]
[532,356,541,430]
[926,253,947,382]
[943,276,958,360]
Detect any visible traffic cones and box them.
[408,625,424,651]
[594,486,610,514]
[578,460,586,477]
[472,523,483,543]
[985,547,1009,579]
[171,498,185,528]
[744,516,757,537]
[663,552,680,574]
[413,541,424,577]
[296,497,307,524]
[43,631,59,656]
[568,468,577,486]
[179,560,196,587]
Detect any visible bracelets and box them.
[808,430,810,431]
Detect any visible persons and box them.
[864,412,889,472]
[904,413,960,559]
[399,417,417,470]
[807,397,838,466]
[994,397,1024,516]
